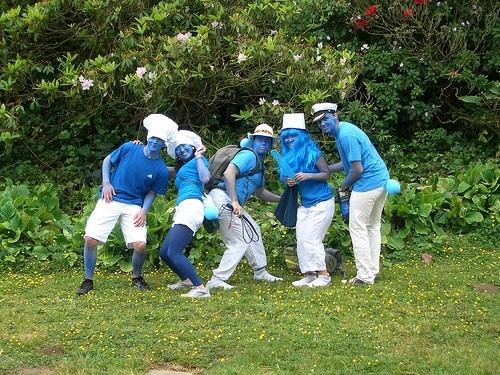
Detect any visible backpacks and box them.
[324,248,342,275]
[205,145,265,195]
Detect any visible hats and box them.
[167,130,202,160]
[144,114,179,141]
[280,113,308,131]
[248,124,280,142]
[310,103,338,122]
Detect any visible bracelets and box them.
[196,155,203,160]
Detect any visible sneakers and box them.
[131,275,151,292]
[77,279,93,296]
[166,280,194,290]
[180,284,211,299]
[253,270,284,282]
[306,273,332,288]
[206,280,237,290]
[292,275,317,288]
[341,277,366,285]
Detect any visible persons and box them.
[275,113,336,288]
[205,124,281,290]
[129,129,212,299]
[76,112,180,296]
[310,102,390,284]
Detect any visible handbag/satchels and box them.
[203,195,220,234]
